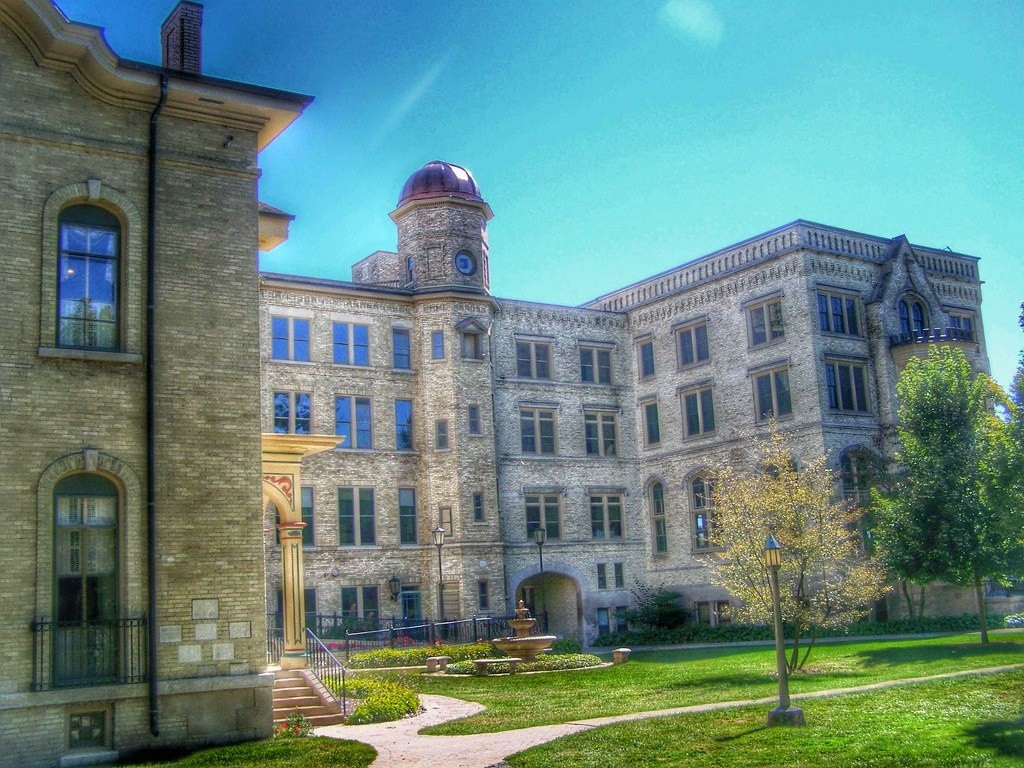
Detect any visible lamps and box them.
[389,575,401,602]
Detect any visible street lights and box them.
[432,524,446,622]
[533,525,549,635]
[763,533,807,728]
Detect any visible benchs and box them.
[427,656,451,673]
[473,658,522,675]
[612,648,631,664]
